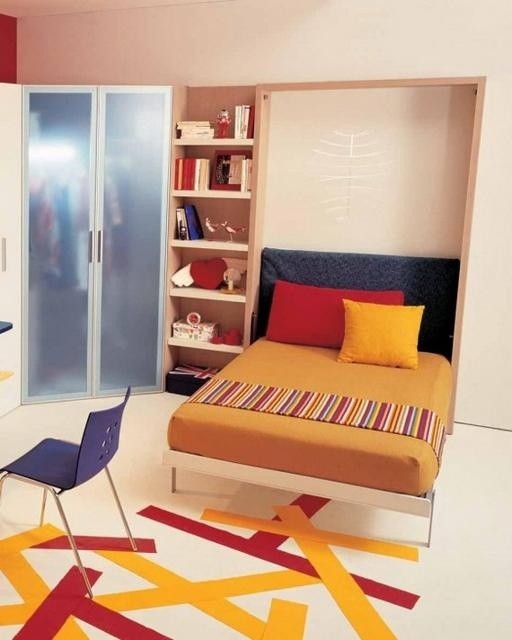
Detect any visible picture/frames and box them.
[211,150,252,190]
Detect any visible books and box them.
[174,158,211,191]
[185,203,204,241]
[241,160,246,192]
[240,106,243,139]
[235,105,241,140]
[245,158,248,192]
[176,207,183,238]
[247,106,255,139]
[181,208,188,239]
[244,105,249,138]
[175,119,216,139]
[248,160,253,192]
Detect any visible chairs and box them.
[1,387,137,597]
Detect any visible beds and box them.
[167,248,460,548]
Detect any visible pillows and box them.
[337,298,425,369]
[266,280,404,349]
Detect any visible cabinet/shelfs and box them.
[22,84,172,405]
[162,86,260,395]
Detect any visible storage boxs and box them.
[172,319,221,343]
[166,365,219,396]
